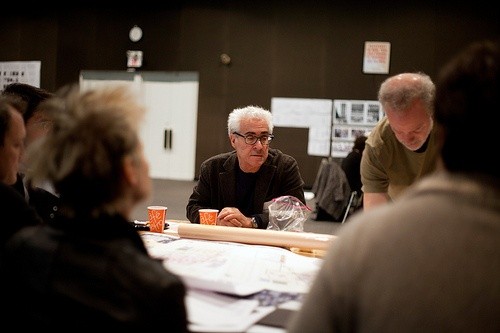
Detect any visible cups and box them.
[147,205,167,232]
[199,209,219,225]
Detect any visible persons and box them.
[0,94,45,244]
[287,40,500,332]
[2,83,56,151]
[185,103,308,228]
[0,82,189,333]
[360,69,450,214]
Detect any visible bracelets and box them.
[250,216,258,228]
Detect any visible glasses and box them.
[231,132,274,145]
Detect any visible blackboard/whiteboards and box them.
[78,72,200,183]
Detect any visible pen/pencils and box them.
[135,226,169,231]
